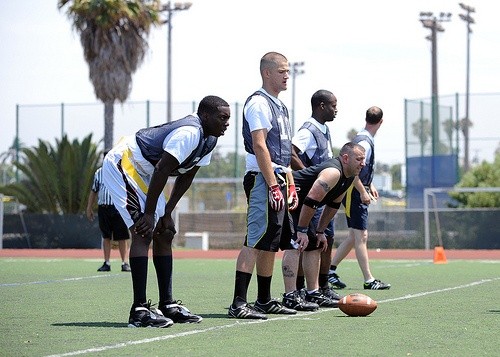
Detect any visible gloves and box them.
[287,184,299,211]
[269,184,284,212]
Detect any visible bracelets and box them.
[297,225,308,233]
[315,229,324,234]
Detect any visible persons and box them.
[290,89,343,300]
[328,106,390,289]
[281,142,366,310]
[87,149,131,273]
[227,52,297,320]
[103,96,230,328]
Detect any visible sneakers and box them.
[319,286,341,300]
[364,280,391,290]
[158,300,203,323]
[282,290,319,310]
[302,288,339,308]
[98,262,110,271]
[228,296,268,319]
[128,299,173,328]
[253,297,297,314]
[122,264,131,271]
[328,273,346,288]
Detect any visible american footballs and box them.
[337,293,378,316]
